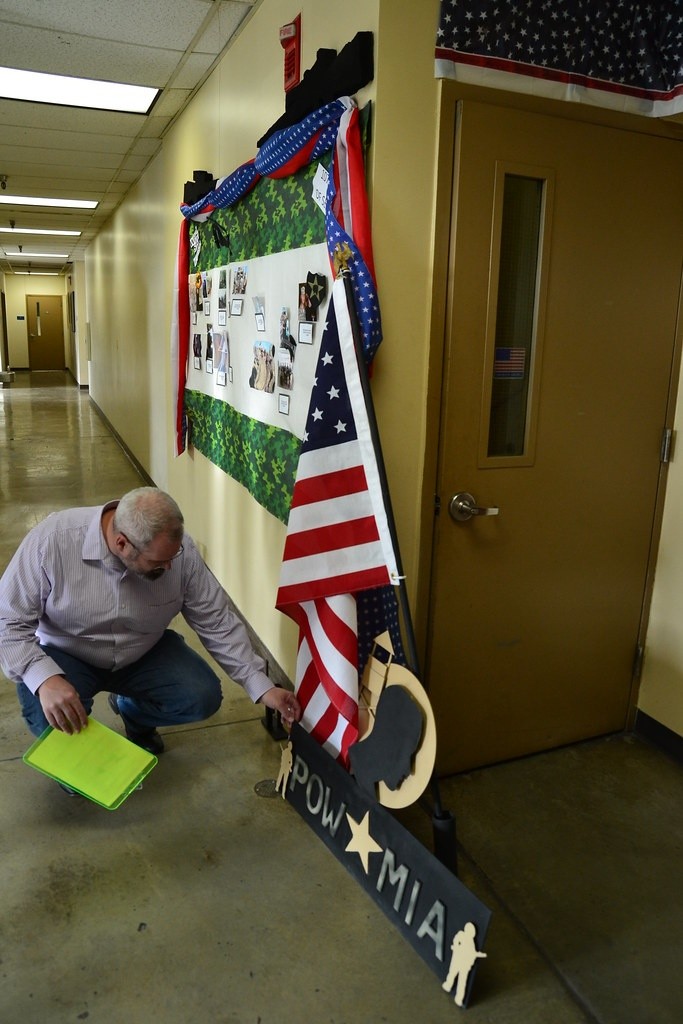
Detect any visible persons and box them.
[0,489,300,790]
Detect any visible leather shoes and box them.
[57,783,79,797]
[108,691,164,754]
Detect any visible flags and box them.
[273,279,404,760]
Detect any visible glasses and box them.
[120,531,184,568]
